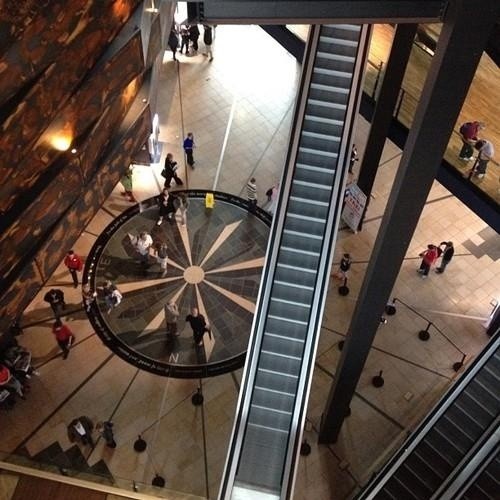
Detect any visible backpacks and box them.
[460,122,472,134]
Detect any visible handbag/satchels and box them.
[161,170,169,178]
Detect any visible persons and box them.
[68,416,95,449]
[348,143,359,175]
[458,120,485,162]
[95,420,116,449]
[247,178,257,214]
[268,183,281,215]
[42,132,212,359]
[416,244,439,279]
[0,326,40,411]
[168,22,213,64]
[436,241,454,274]
[335,254,353,282]
[475,139,495,179]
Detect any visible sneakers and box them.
[469,157,475,161]
[459,156,469,161]
[478,173,483,179]
[422,275,426,279]
[417,269,423,272]
[156,220,162,225]
